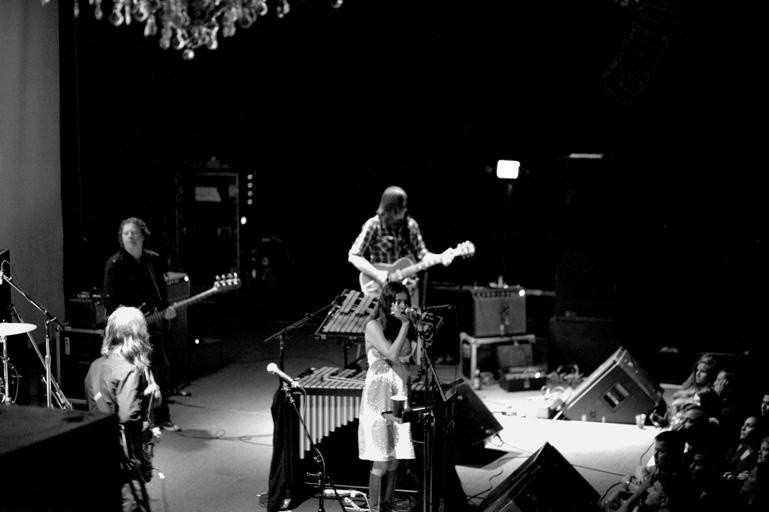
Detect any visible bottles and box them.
[473,369,482,390]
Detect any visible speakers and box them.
[478,441,601,512]
[563,347,665,424]
[549,317,606,377]
[463,285,527,336]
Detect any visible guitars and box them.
[359,241,476,298]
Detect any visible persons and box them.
[85,303,153,508]
[102,217,183,435]
[349,186,454,320]
[357,286,427,512]
[601,338,769,511]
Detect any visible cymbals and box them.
[0,322,38,337]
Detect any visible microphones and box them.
[404,307,415,314]
[264,362,299,391]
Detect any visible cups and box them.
[635,412,646,429]
